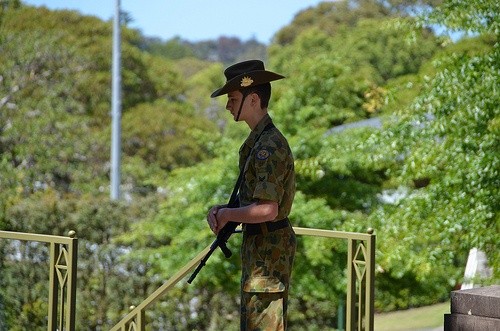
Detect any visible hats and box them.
[211,60,285,98]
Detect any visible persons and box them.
[207,59,296,331]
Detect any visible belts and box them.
[244,218,289,233]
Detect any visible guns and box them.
[188,193,242,284]
